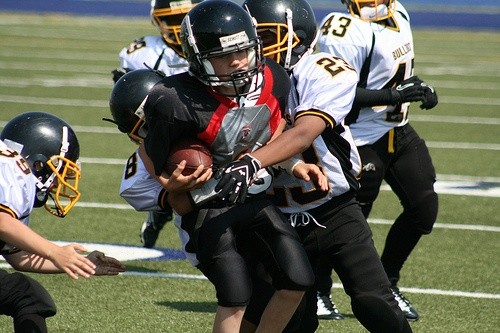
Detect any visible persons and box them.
[0,112,128,333]
[108,0,414,333]
[311,0,440,322]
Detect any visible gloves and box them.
[214,153,263,204]
[396,76,438,110]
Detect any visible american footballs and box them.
[165,139,212,179]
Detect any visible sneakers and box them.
[140,210,172,246]
[391,285,419,320]
[316,292,343,320]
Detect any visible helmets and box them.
[180,0,265,97]
[242,0,316,71]
[151,0,202,45]
[109,69,167,143]
[1,111,80,207]
